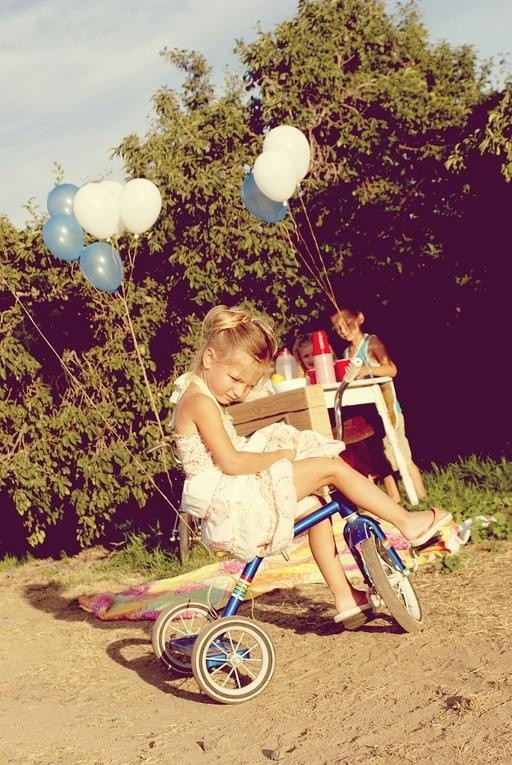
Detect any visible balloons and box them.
[237,126,312,226]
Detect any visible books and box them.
[42,179,161,294]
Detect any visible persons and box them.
[169,304,452,629]
[329,302,427,501]
[295,331,335,370]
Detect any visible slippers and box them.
[332,592,385,623]
[410,508,453,548]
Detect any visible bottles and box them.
[275,347,299,380]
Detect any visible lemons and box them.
[271,372,286,382]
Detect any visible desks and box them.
[228,376,420,509]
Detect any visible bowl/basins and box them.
[270,377,306,395]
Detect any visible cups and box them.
[307,330,351,385]
